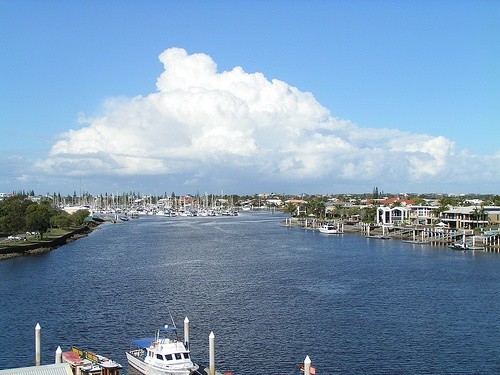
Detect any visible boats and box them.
[319,222,344,234]
[61,346,123,375]
[125,315,223,375]
